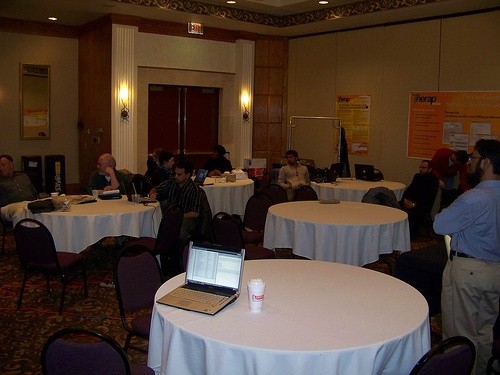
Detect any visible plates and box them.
[319,200,340,204]
[128,198,158,203]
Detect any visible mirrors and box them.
[20,61,50,141]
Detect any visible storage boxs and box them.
[244,157,280,179]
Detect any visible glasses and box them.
[468,155,481,161]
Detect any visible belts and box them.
[449,249,474,261]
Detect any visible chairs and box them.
[183,234,213,271]
[114,243,164,354]
[361,185,400,267]
[128,207,183,274]
[13,218,89,315]
[40,327,156,375]
[212,183,318,259]
[395,244,500,375]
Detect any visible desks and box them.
[191,175,255,222]
[1,195,163,259]
[147,259,430,375]
[309,178,405,203]
[263,200,411,267]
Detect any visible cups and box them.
[247,279,265,311]
[215,176,225,183]
[92,190,98,198]
[132,194,140,207]
[231,170,237,174]
[224,172,230,176]
[51,193,58,197]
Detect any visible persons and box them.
[0,154,38,207]
[143,151,200,238]
[201,144,233,173]
[434,139,500,375]
[395,148,470,241]
[86,154,134,195]
[277,150,311,201]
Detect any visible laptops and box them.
[156,241,246,316]
[355,164,381,182]
[194,169,208,185]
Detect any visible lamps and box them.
[118,86,131,122]
[241,92,251,124]
